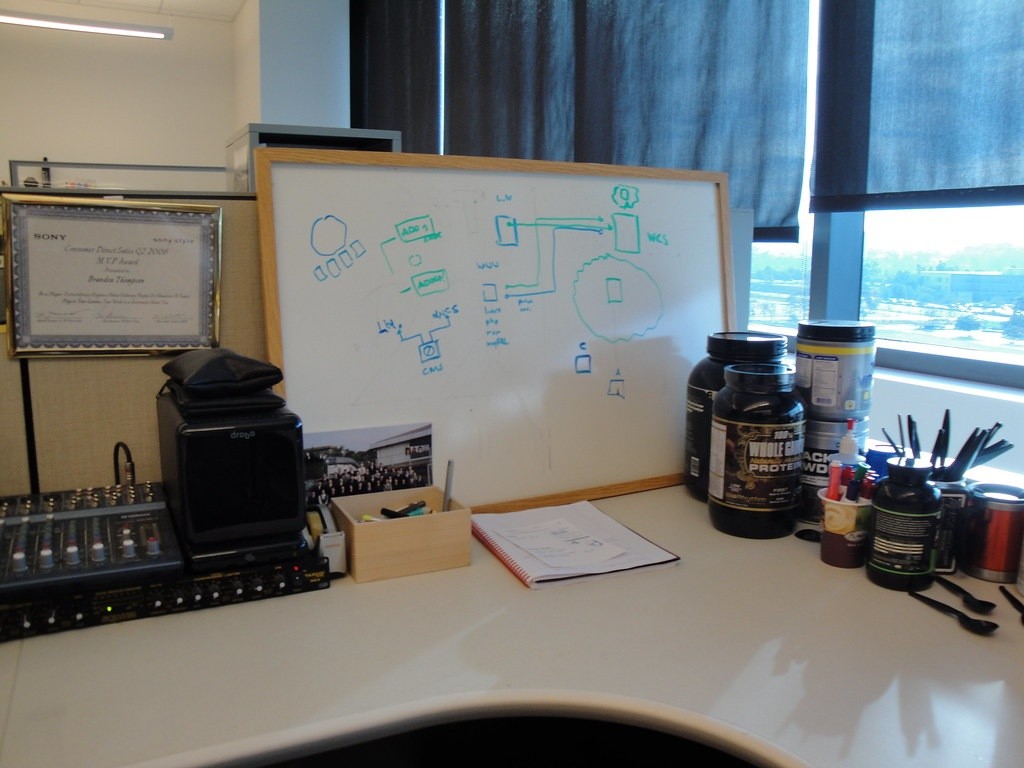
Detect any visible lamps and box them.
[0,9,173,40]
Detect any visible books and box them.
[471,498,680,591]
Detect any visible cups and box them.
[927,457,966,574]
[816,485,873,568]
[958,483,1024,583]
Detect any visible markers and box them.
[826,460,871,504]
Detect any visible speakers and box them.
[156,392,307,547]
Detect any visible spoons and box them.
[909,590,999,634]
[934,575,997,614]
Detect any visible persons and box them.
[307,446,429,509]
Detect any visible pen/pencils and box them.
[442,459,454,512]
[882,407,1015,481]
[397,501,426,514]
[380,508,409,519]
[362,514,380,521]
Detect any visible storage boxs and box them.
[330,486,471,585]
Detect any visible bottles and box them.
[839,418,858,457]
[684,331,790,505]
[708,362,808,540]
[865,457,943,592]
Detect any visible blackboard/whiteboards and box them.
[254,146,738,514]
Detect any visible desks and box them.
[0,486,1024,768]
